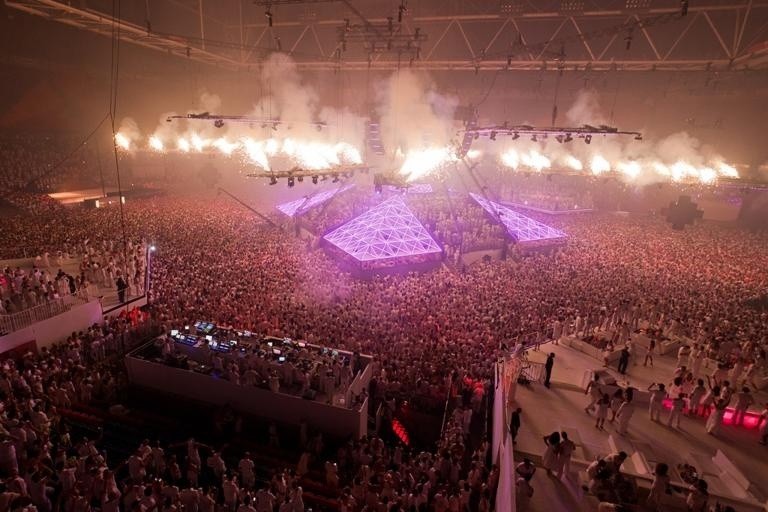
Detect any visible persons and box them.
[0,132,768,512]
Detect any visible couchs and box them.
[562,333,768,512]
